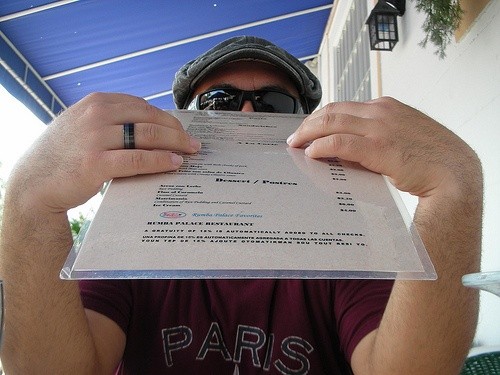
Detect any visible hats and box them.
[172,36,322,115]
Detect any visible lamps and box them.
[364,0,406,51]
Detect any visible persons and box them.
[0,36,484,375]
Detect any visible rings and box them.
[122,123,134,150]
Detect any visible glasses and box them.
[187,88,303,114]
